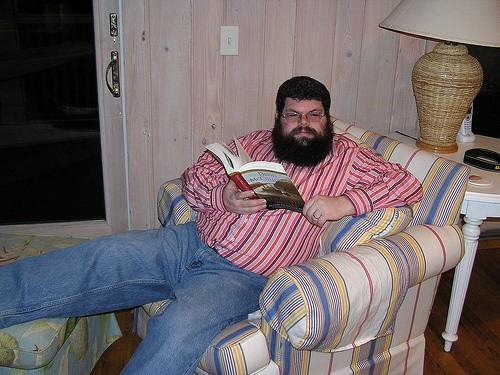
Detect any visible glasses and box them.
[281,109,327,122]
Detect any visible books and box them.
[205,134,306,212]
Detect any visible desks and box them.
[393,129,500,352]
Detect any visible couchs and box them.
[132,115,471,375]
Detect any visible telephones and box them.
[456,102,475,143]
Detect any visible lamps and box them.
[379,0,500,154]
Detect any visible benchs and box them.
[0,232,122,375]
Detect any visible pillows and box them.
[318,204,413,257]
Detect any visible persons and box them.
[0,75,425,375]
